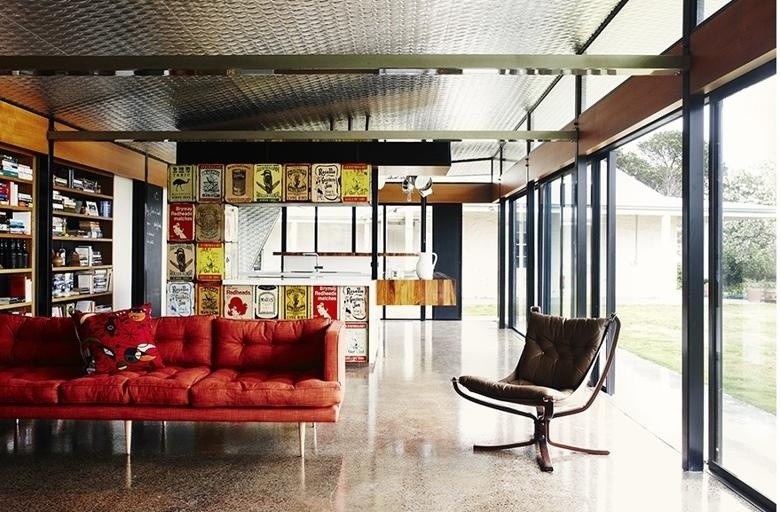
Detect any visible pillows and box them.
[69,303,168,375]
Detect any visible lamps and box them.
[401,176,434,197]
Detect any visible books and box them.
[52,169,113,318]
[0,154,33,236]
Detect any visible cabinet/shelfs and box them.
[224,243,239,280]
[39,155,113,318]
[0,141,37,316]
[223,203,240,243]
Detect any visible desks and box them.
[376,270,457,321]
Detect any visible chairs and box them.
[451,306,620,472]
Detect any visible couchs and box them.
[0,312,348,457]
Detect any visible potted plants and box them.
[742,277,767,303]
[763,281,776,301]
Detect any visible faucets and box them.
[302,252,324,273]
